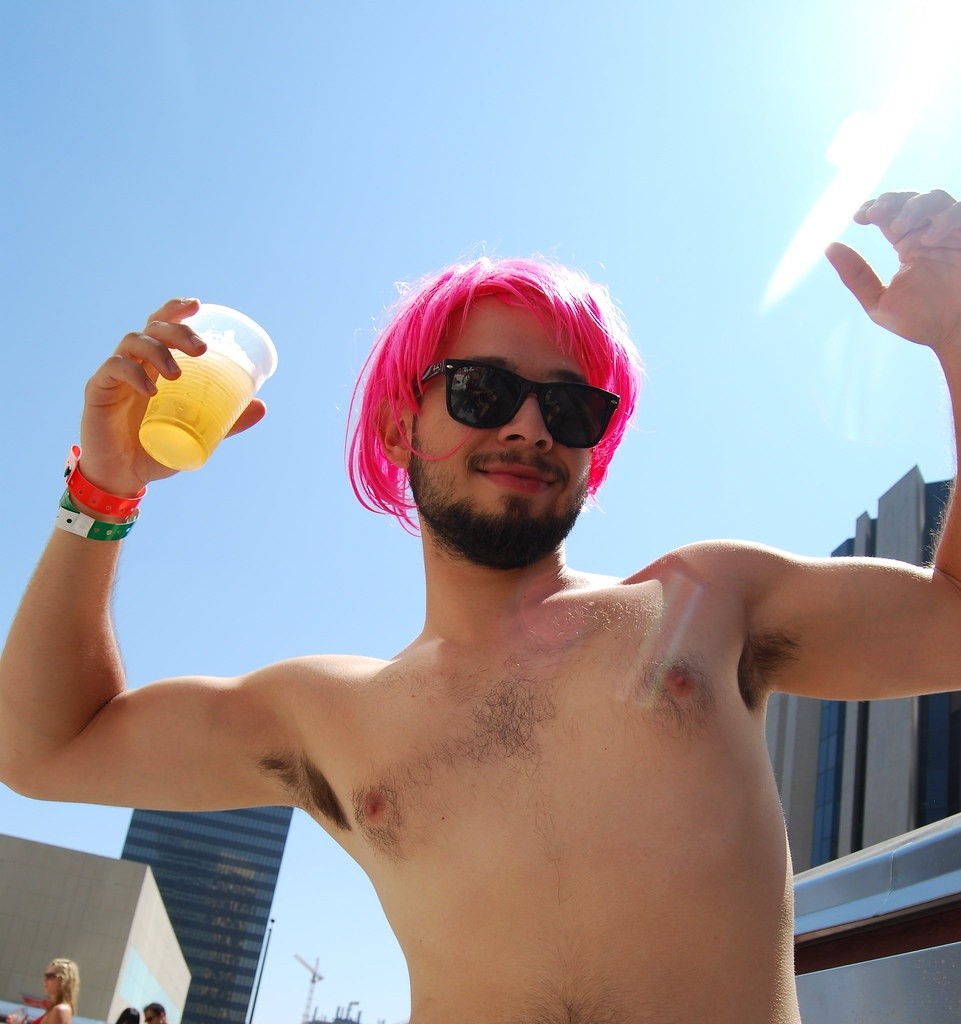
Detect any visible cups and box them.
[138,303,279,472]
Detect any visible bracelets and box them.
[54,443,148,544]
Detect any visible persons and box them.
[27,957,82,1024]
[1,186,961,1024]
[115,1002,169,1024]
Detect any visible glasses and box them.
[43,972,57,980]
[412,358,620,449]
[145,1015,158,1022]
